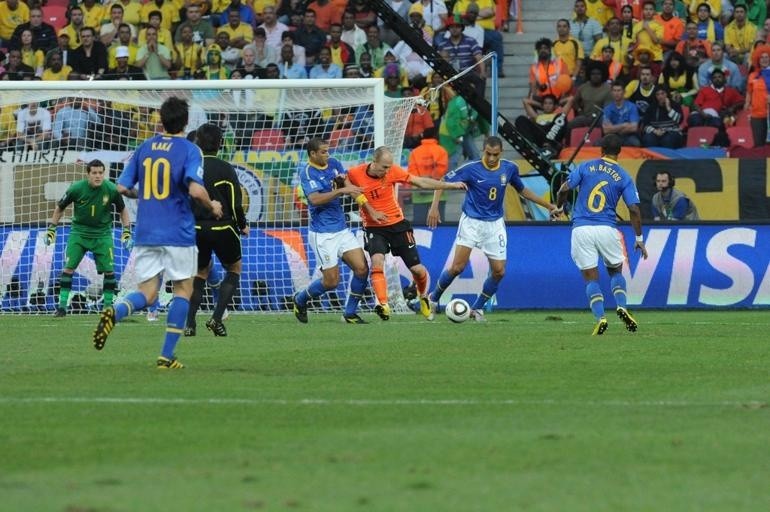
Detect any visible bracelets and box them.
[635,234,643,241]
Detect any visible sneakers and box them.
[416,288,438,322]
[616,306,638,333]
[291,290,309,324]
[205,317,228,337]
[469,307,487,322]
[341,313,371,325]
[156,356,187,369]
[53,305,67,318]
[374,303,390,321]
[146,309,160,322]
[591,316,609,336]
[213,305,230,321]
[91,305,116,352]
[183,326,197,336]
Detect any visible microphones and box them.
[663,185,671,189]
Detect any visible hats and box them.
[407,2,425,17]
[442,14,469,29]
[113,45,131,60]
[343,64,361,74]
[207,43,222,54]
[383,62,402,84]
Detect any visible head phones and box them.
[653,169,674,187]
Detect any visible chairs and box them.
[569,103,756,157]
[40,1,355,151]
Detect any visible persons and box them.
[291,137,391,324]
[342,148,470,319]
[0,1,769,223]
[94,96,223,368]
[551,134,648,336]
[184,122,249,336]
[38,157,132,316]
[426,136,561,323]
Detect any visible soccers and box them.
[446,298,470,323]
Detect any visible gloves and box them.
[120,225,133,252]
[43,221,58,246]
